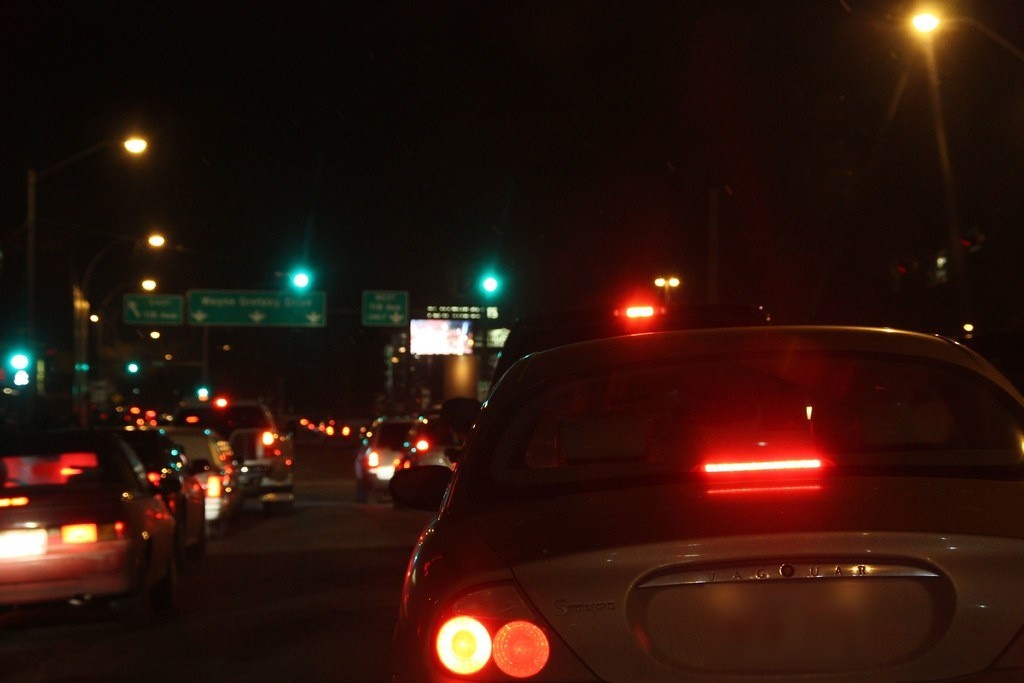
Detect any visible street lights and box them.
[23,131,212,408]
[654,277,681,305]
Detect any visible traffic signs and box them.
[361,290,408,327]
[187,290,327,327]
[123,292,185,325]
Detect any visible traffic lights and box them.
[626,306,654,319]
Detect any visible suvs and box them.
[170,400,293,515]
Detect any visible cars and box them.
[1,430,177,606]
[121,427,213,566]
[386,324,1024,682]
[355,418,458,507]
[161,427,241,523]
